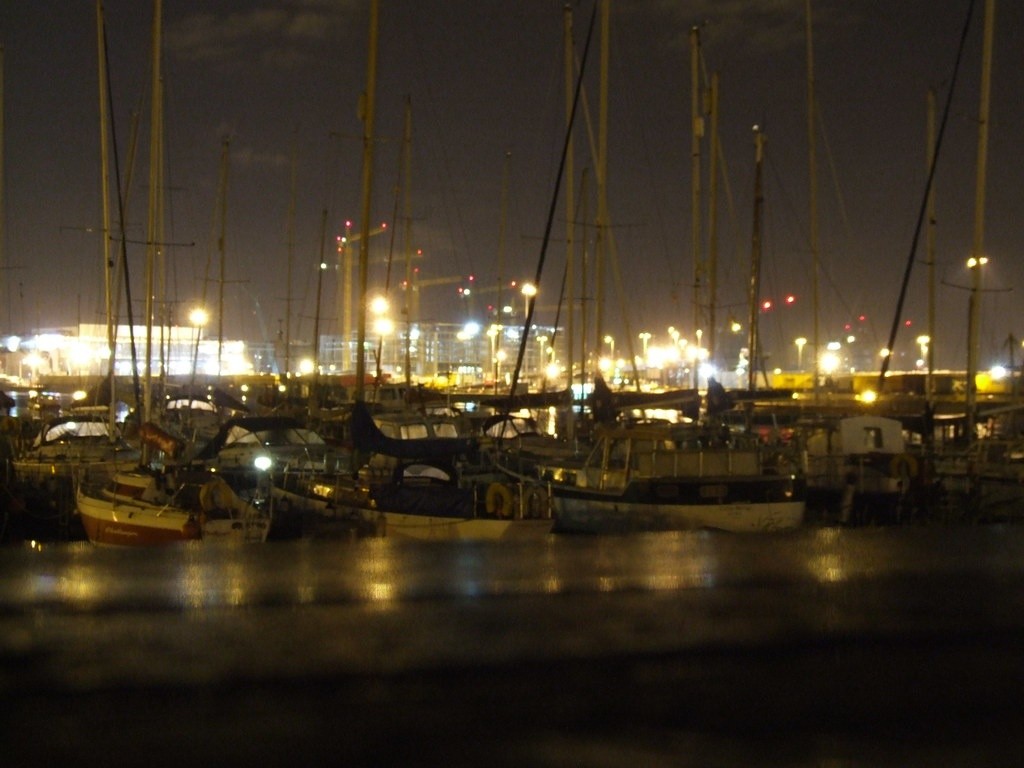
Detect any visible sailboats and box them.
[0,2,1017,551]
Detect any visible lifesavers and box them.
[199,479,234,513]
[888,452,920,480]
[522,484,549,519]
[488,482,515,515]
